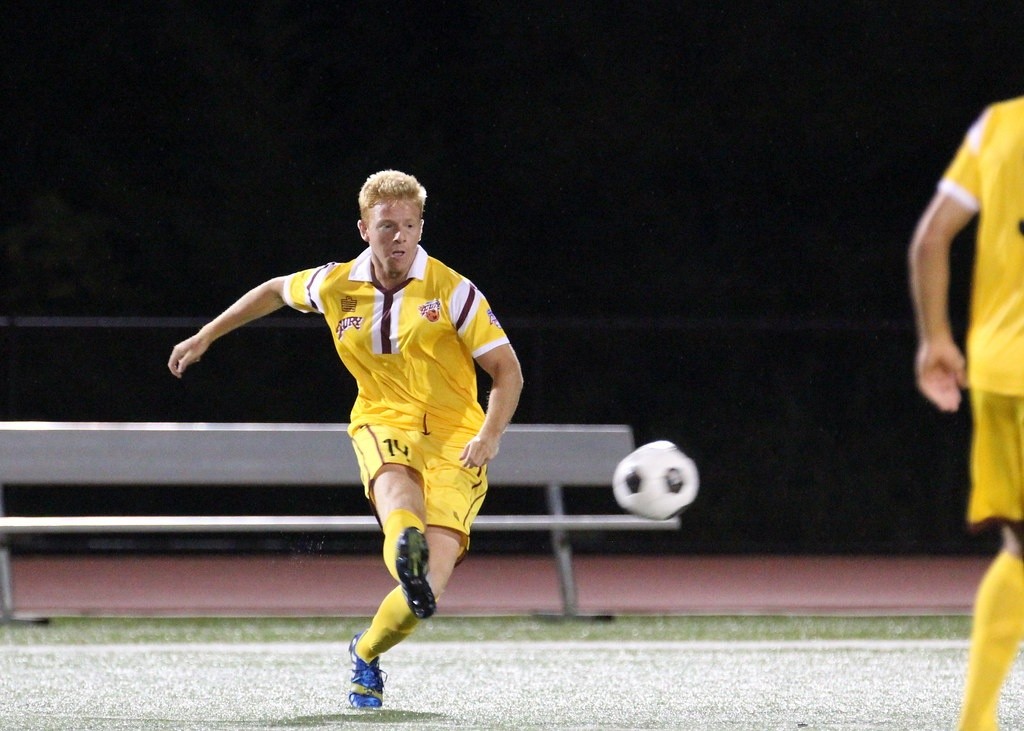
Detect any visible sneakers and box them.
[396,528,437,619]
[348,634,387,709]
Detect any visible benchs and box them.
[0,420,680,627]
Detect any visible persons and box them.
[907,93,1024,731]
[167,169,523,708]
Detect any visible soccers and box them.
[612,440,700,521]
